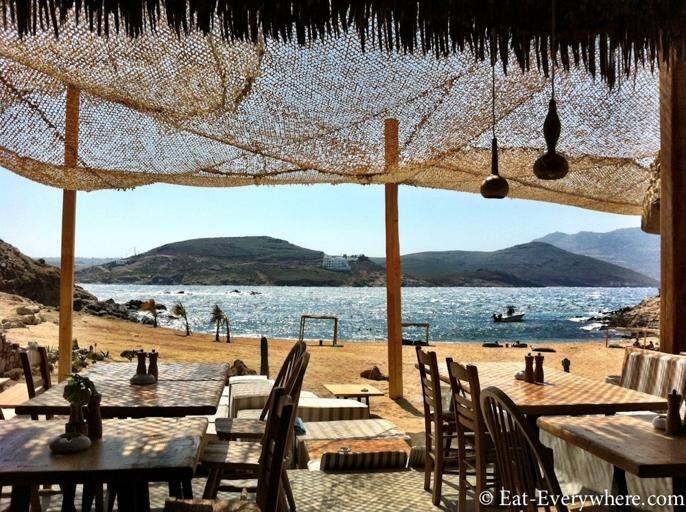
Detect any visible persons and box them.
[639,341,654,349]
[633,338,641,347]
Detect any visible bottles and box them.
[665,388,682,434]
[70,391,104,439]
[137,348,158,380]
[524,352,545,382]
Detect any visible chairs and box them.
[199,340,311,512]
[619,344,684,406]
[18,345,58,420]
[415,346,570,511]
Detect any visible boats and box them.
[492,304,524,324]
[403,337,430,346]
[480,338,557,355]
[164,288,262,298]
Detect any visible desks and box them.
[414,361,686,512]
[0,361,230,511]
[322,384,384,416]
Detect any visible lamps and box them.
[479,6,568,200]
